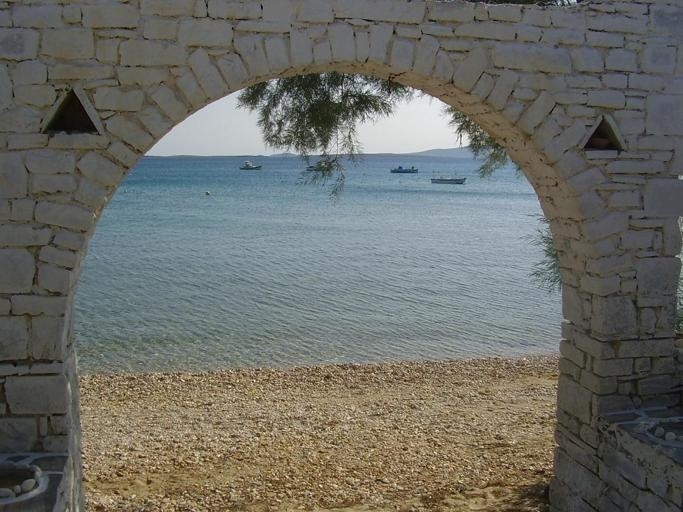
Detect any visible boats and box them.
[430,176,465,184]
[239,161,261,170]
[305,165,331,170]
[390,166,417,173]
